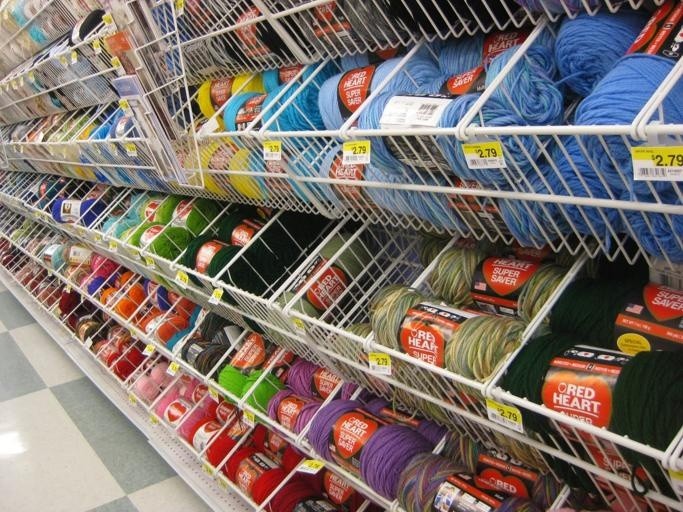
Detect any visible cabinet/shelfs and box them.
[0,0,680,511]
